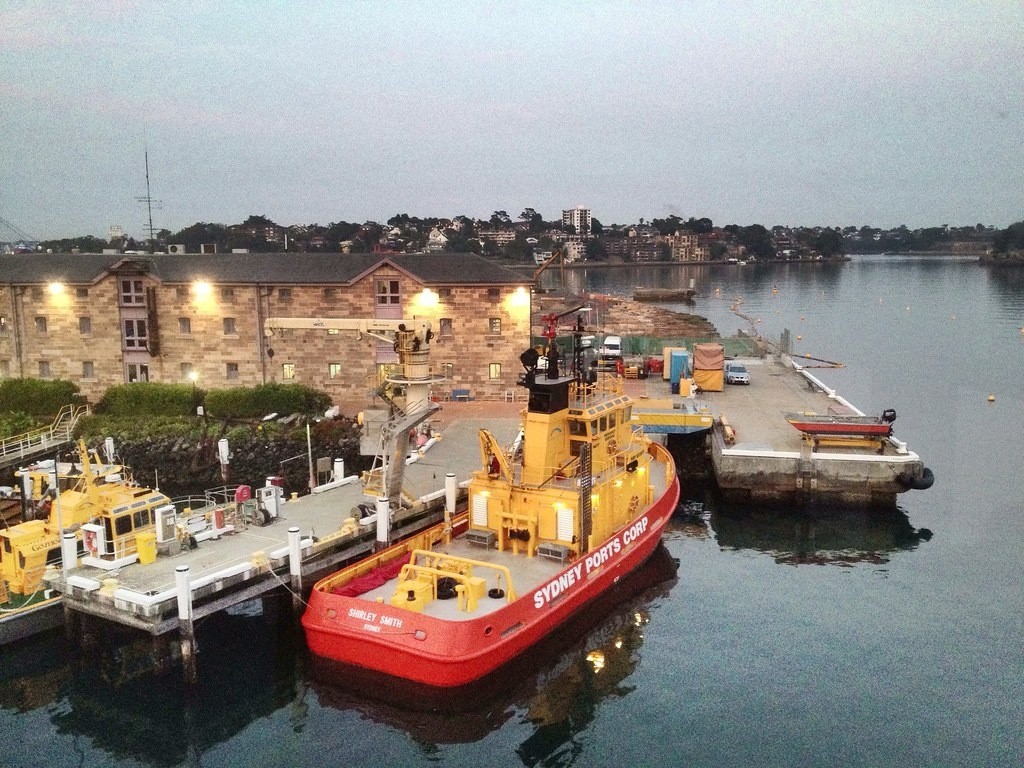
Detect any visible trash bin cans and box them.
[673,383,679,394]
[638,367,644,379]
[133,532,158,564]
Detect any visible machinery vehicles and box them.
[531,251,564,293]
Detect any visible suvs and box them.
[725,362,750,385]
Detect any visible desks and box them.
[538,542,570,567]
[457,394,468,403]
[465,529,495,551]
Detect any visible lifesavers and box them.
[86,531,97,552]
[435,577,457,600]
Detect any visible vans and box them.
[601,336,622,360]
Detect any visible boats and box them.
[780,409,897,436]
[300,313,681,686]
[633,279,697,301]
[0,437,141,530]
[0,436,251,645]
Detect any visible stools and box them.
[432,396,439,402]
[444,396,450,402]
